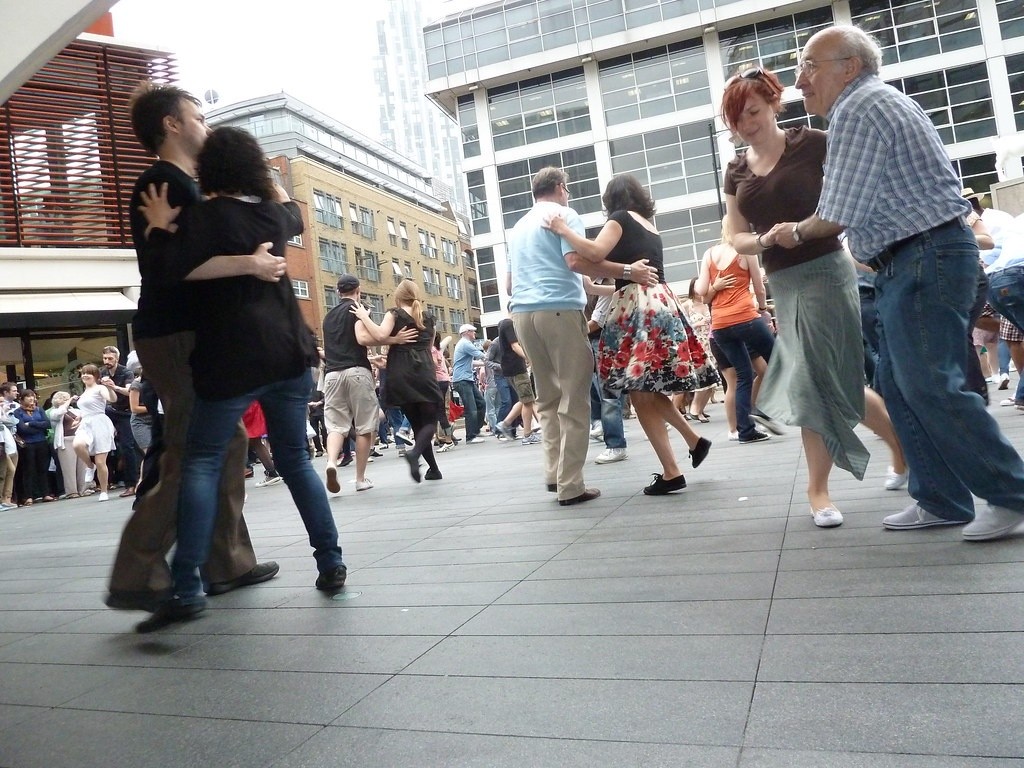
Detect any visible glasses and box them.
[722,66,764,92]
[793,56,852,78]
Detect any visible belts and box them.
[989,267,1024,277]
[867,217,972,272]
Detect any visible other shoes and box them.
[0,485,116,512]
[119,487,136,497]
[369,422,542,461]
[135,595,205,635]
[255,471,280,487]
[315,567,346,592]
[424,468,442,480]
[547,483,558,492]
[404,451,420,483]
[315,450,324,458]
[559,489,601,506]
[337,456,352,467]
[748,410,785,435]
[103,589,167,613]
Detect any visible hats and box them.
[961,188,985,202]
[337,274,360,293]
[459,324,478,335]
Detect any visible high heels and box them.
[711,398,718,404]
[688,409,711,423]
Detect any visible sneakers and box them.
[595,446,628,465]
[327,464,340,493]
[643,472,687,495]
[356,477,373,491]
[811,501,844,526]
[738,428,772,444]
[85,462,96,483]
[1015,405,1024,415]
[961,503,1022,540]
[999,373,1009,391]
[202,560,280,595]
[590,419,605,442]
[688,436,712,469]
[882,503,970,530]
[98,492,108,502]
[728,430,738,441]
[884,466,912,491]
[1000,393,1018,405]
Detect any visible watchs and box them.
[792,223,806,245]
[623,265,632,281]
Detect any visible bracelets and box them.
[711,285,717,292]
[758,307,767,311]
[756,231,773,250]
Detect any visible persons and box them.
[504,166,659,507]
[724,66,911,527]
[539,172,721,496]
[103,81,281,611]
[768,25,1024,542]
[0,186,1024,512]
[134,127,348,633]
